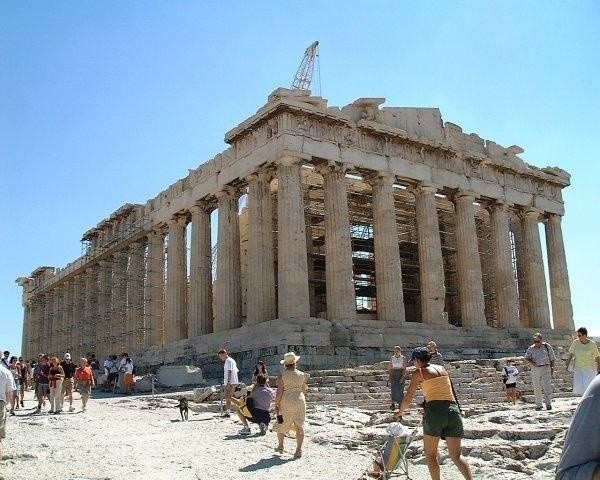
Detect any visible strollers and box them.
[358,407,425,479]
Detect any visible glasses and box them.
[5,355,23,361]
[534,340,540,343]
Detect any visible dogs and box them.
[175,397,191,422]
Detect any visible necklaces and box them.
[395,355,400,360]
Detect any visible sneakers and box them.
[259,422,266,435]
[239,426,251,435]
[69,406,75,411]
[293,450,303,459]
[20,400,24,407]
[10,408,15,416]
[81,405,87,411]
[220,413,231,419]
[31,405,64,416]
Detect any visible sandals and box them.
[547,404,551,410]
[535,405,543,411]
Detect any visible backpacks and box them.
[237,383,256,421]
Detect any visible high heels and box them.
[273,445,284,454]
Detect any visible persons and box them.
[523,332,556,411]
[386,345,407,411]
[395,346,474,480]
[273,351,311,460]
[427,341,444,365]
[565,327,600,396]
[0,350,136,440]
[251,361,270,388]
[218,348,239,419]
[236,375,275,435]
[501,358,519,405]
[553,371,600,479]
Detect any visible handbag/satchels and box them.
[503,378,506,383]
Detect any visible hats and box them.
[408,346,433,364]
[63,352,72,360]
[279,351,301,365]
[385,421,405,437]
